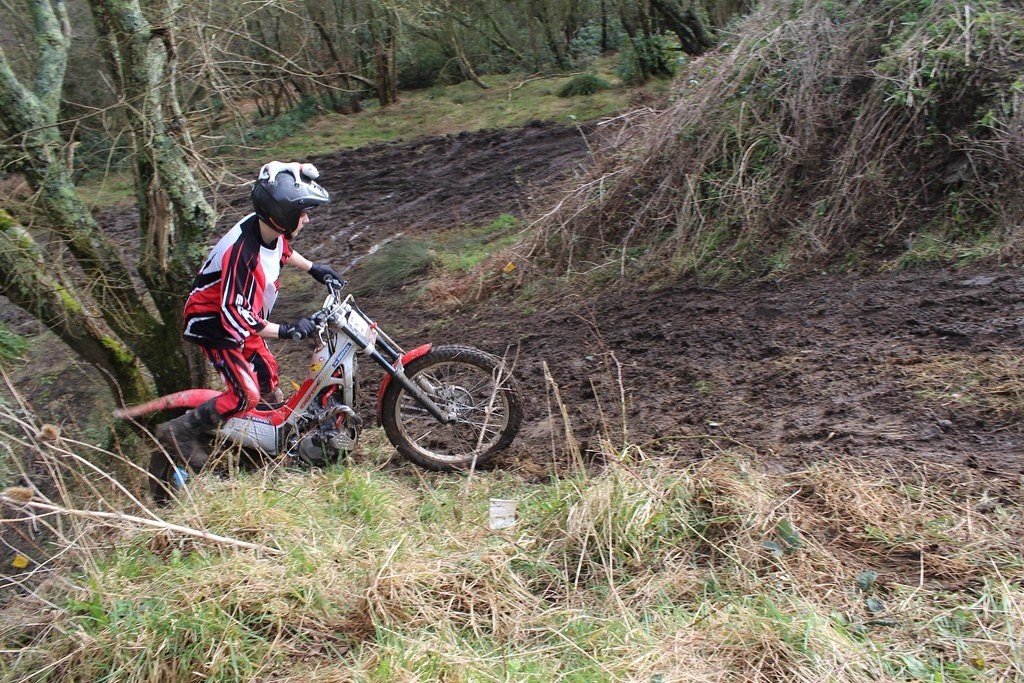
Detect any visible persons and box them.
[157,160,344,474]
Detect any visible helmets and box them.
[251,170,332,231]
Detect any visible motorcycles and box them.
[113,275,525,507]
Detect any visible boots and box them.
[158,396,226,468]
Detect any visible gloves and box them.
[307,261,344,288]
[278,317,318,341]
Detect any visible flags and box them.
[504,262,515,273]
[172,467,188,487]
